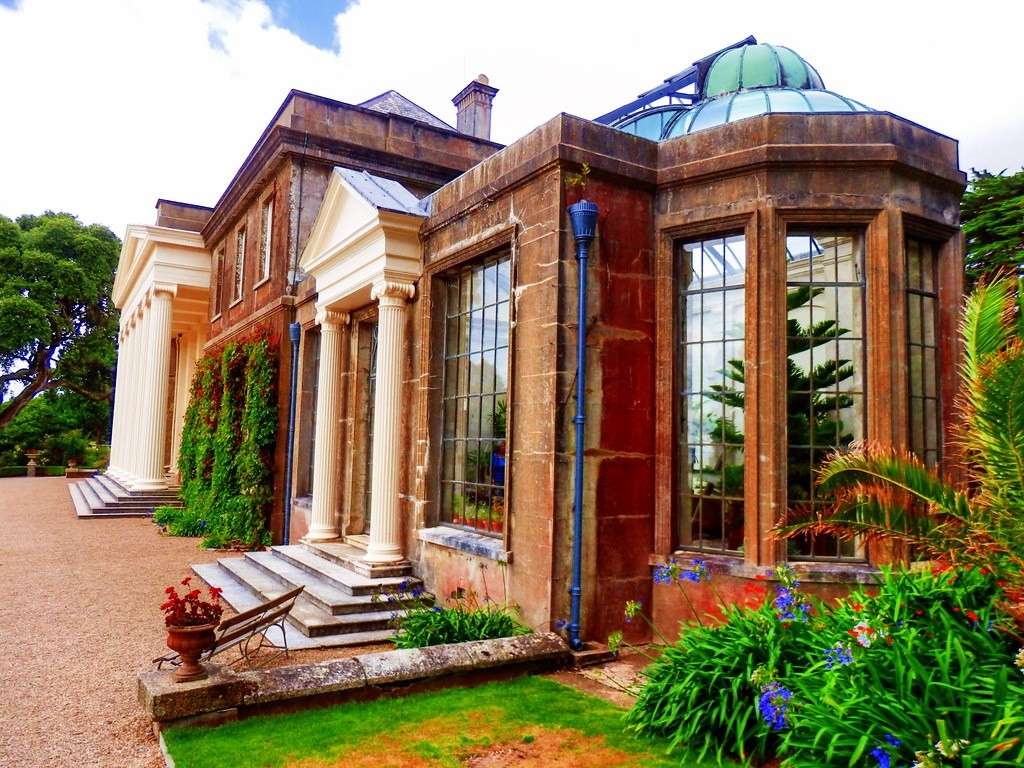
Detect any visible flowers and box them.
[68,457,77,462]
[26,449,38,455]
[160,578,223,627]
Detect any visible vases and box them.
[68,461,77,469]
[26,454,37,464]
[166,622,219,682]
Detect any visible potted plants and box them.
[453,493,503,535]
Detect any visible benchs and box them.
[151,584,305,671]
